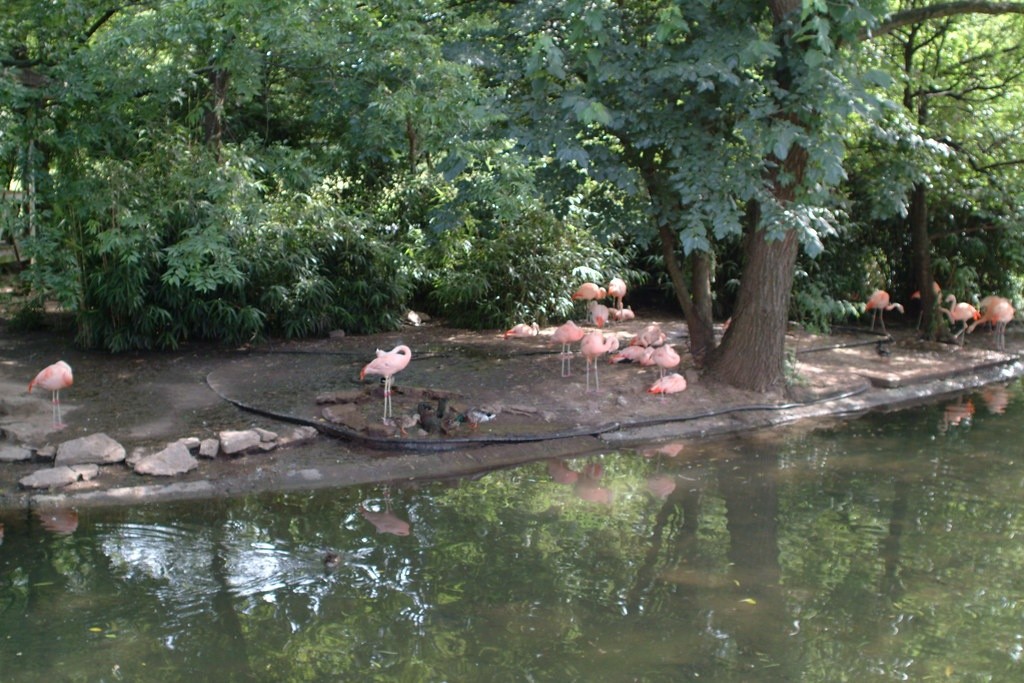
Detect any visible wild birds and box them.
[361,345,412,417]
[29,361,74,429]
[967,292,1016,350]
[551,279,687,405]
[504,322,540,338]
[866,290,904,335]
[912,281,980,347]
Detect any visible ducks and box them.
[393,398,496,437]
